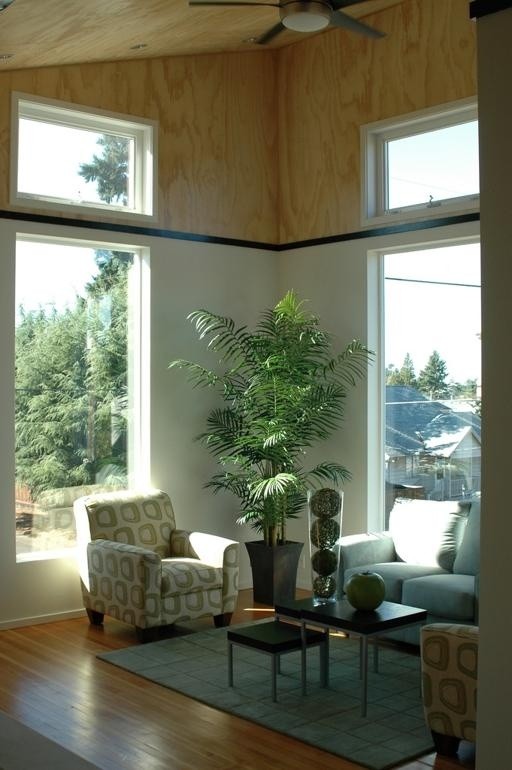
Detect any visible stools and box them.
[228,622,327,702]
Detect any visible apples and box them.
[346,570,384,612]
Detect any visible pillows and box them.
[389,498,481,575]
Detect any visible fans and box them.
[189,1,395,46]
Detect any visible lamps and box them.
[280,4,333,34]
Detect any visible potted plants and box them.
[166,288,377,603]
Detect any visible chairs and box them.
[70,487,241,642]
[419,623,476,762]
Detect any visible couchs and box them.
[324,496,481,651]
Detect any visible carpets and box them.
[96,616,440,766]
